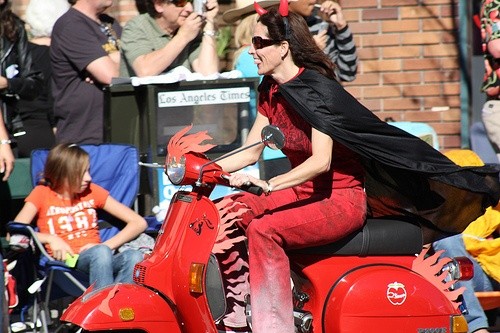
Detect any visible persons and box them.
[25,0,71,158]
[288,0,357,84]
[13,144,147,290]
[215,0,500,333]
[0,0,44,238]
[222,0,292,181]
[432,228,500,333]
[49,0,138,145]
[480,0,500,160]
[119,0,219,78]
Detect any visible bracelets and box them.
[202,30,216,36]
[265,180,272,193]
[0,140,12,145]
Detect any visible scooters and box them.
[58,125,474,333]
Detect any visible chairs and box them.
[6,143,162,333]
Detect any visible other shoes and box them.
[214,323,252,332]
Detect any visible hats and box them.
[223,0,296,22]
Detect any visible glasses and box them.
[251,35,289,49]
[166,0,193,6]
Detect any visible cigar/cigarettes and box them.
[314,4,322,8]
